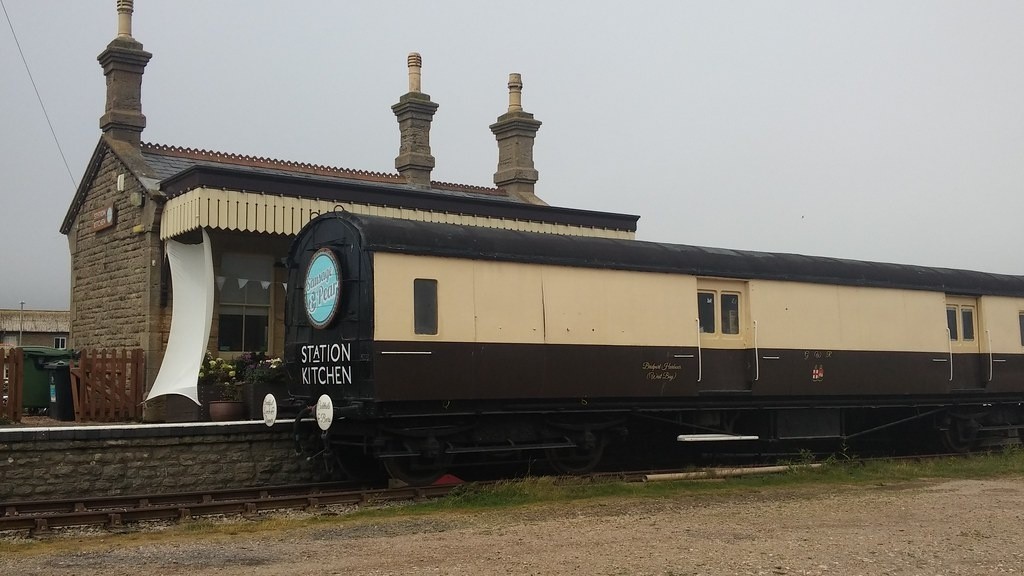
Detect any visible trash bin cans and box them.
[14,347,80,423]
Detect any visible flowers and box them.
[198,350,286,402]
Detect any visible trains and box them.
[262,204,1024,489]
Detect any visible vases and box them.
[235,384,283,418]
[209,401,243,422]
